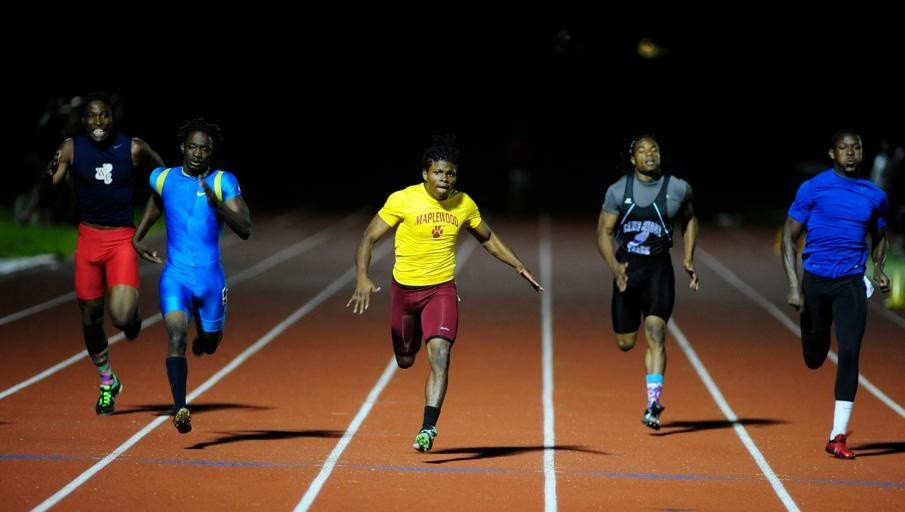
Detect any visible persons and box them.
[596,134,700,430]
[131,117,250,433]
[781,131,890,459]
[346,144,545,453]
[48,99,166,415]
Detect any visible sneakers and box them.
[96,373,124,416]
[192,335,205,357]
[641,400,665,431]
[412,427,438,453]
[825,432,855,459]
[173,407,192,433]
[122,304,141,342]
